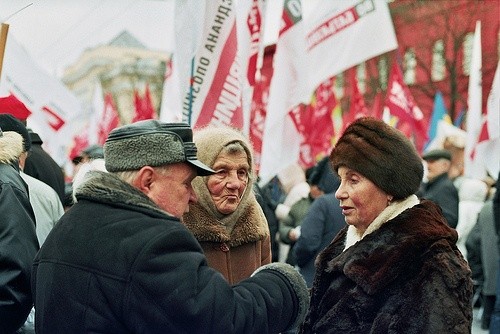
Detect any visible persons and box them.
[32,119,310,334]
[253,157,347,291]
[305,116,473,334]
[183,125,272,285]
[414,126,500,334]
[1,113,105,334]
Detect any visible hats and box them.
[305,156,340,192]
[0,95,31,120]
[105,118,217,177]
[83,144,104,159]
[423,150,452,162]
[330,116,424,200]
[0,113,31,151]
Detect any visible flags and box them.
[1,1,500,189]
